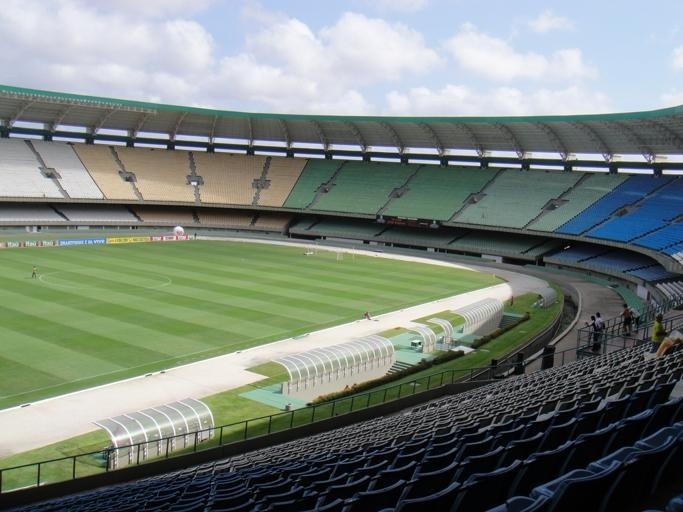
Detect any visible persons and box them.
[31,266,36,279]
[586,303,682,357]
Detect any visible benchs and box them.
[656,244,683,304]
